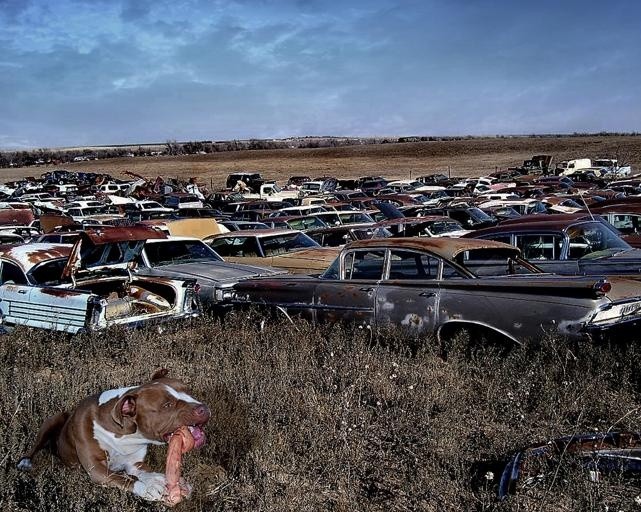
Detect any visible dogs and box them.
[15,368,212,503]
